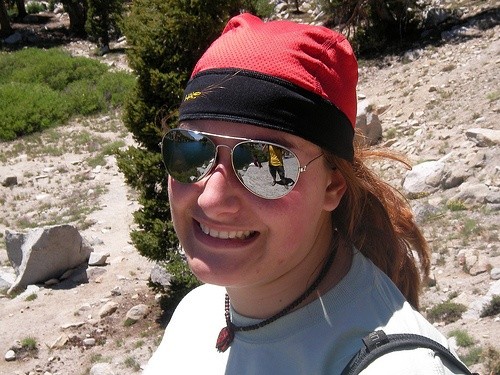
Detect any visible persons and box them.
[141,14,473,375]
[262,144,288,189]
[250,144,262,168]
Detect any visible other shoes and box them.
[284,184,288,189]
[272,181,276,186]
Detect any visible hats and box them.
[176,12,359,161]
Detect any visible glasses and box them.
[158,128,324,200]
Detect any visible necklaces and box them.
[215,231,341,352]
